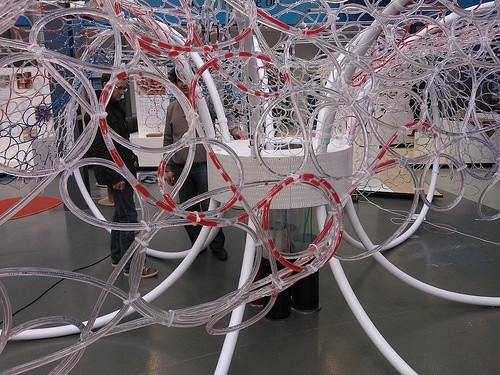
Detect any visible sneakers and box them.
[123,265,159,278]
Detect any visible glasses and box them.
[114,85,128,92]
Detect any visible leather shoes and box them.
[210,246,228,261]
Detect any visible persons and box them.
[162,68,249,261]
[84,71,159,279]
